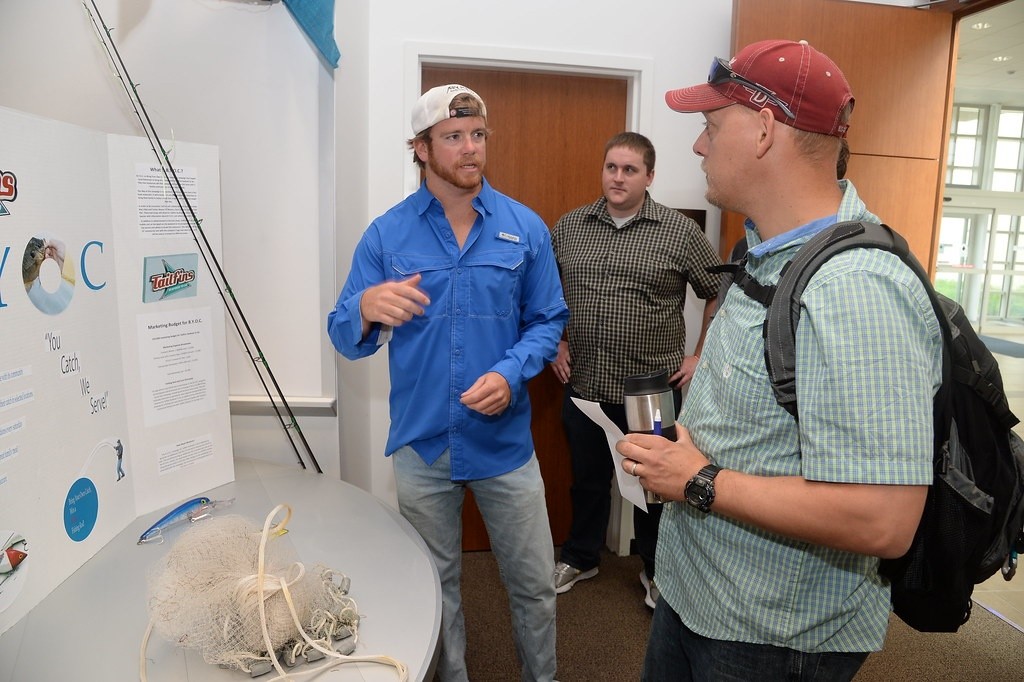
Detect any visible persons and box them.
[615,37,945,682]
[327,81,570,682]
[550,130,725,611]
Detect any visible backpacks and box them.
[699,220,1024,634]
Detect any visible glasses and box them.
[707,55,796,121]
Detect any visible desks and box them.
[1,458,442,682]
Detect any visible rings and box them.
[631,463,637,476]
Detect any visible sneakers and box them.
[640,569,661,608]
[554,562,600,593]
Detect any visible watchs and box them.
[684,464,724,514]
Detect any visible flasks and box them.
[623,368,678,504]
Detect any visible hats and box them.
[665,39,856,138]
[412,83,486,135]
